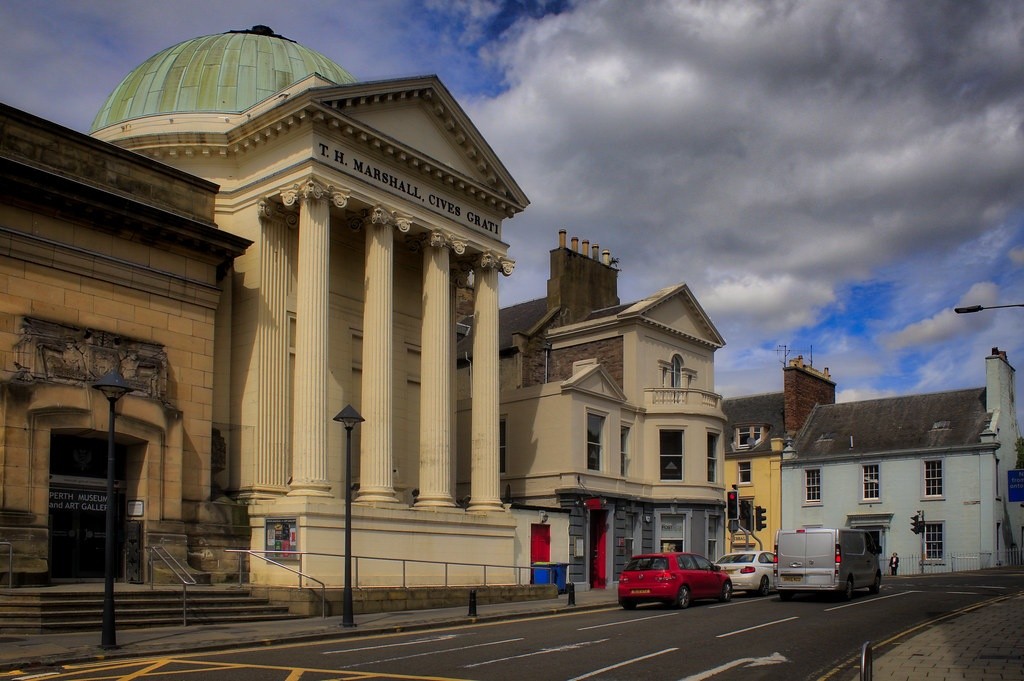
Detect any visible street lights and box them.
[333,403,367,628]
[90,367,135,651]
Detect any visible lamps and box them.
[351,481,360,493]
[541,513,549,523]
[412,488,419,503]
[464,495,471,511]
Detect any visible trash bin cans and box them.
[533,562,557,584]
[552,562,569,591]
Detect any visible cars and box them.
[713,550,779,598]
[617,551,733,610]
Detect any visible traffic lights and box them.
[740,499,750,521]
[726,491,739,521]
[918,521,925,533]
[910,515,920,535]
[756,505,767,531]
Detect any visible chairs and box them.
[653,560,665,568]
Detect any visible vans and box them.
[772,527,882,601]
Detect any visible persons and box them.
[888,553,899,576]
